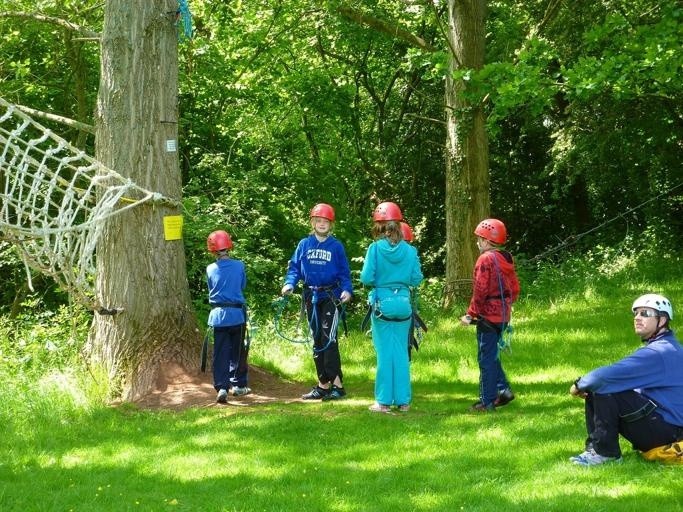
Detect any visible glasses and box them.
[633,309,658,317]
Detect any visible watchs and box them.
[465,315,474,321]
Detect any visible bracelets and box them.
[574,377,581,390]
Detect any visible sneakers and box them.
[397,405,410,411]
[572,448,623,467]
[494,388,515,407]
[216,389,227,403]
[569,443,593,462]
[466,401,495,412]
[232,385,252,396]
[369,404,392,412]
[322,385,346,402]
[302,386,330,399]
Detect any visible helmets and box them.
[631,294,673,320]
[474,218,507,244]
[207,230,233,252]
[372,201,403,222]
[400,222,412,241]
[310,203,335,222]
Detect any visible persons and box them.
[461,219,520,414]
[398,222,414,364]
[567,294,683,470]
[205,230,253,403]
[361,202,425,415]
[281,203,354,403]
